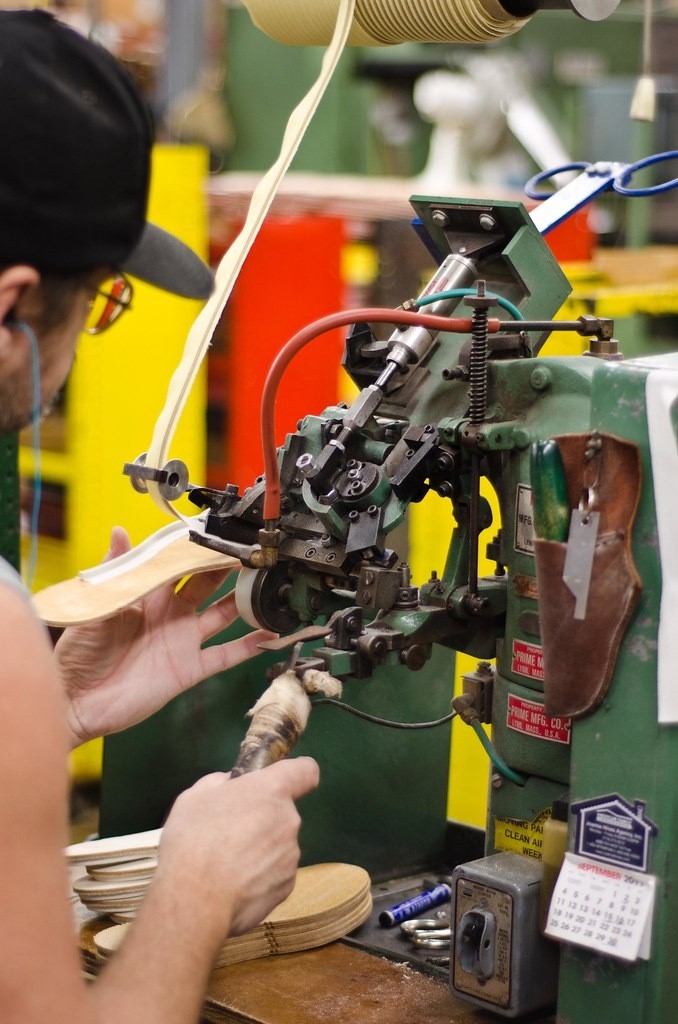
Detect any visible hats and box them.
[0,9,214,300]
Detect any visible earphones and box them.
[2,307,20,329]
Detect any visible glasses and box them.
[49,271,135,336]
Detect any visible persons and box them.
[0,10,317,1024]
